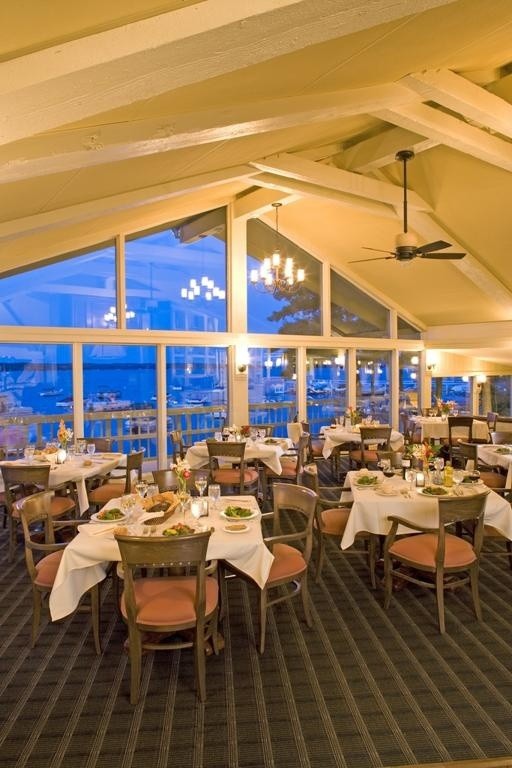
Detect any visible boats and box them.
[95,390,122,400]
[126,415,172,432]
[54,396,90,407]
[40,387,63,397]
[150,391,173,401]
[306,382,333,397]
[185,395,208,405]
[87,394,133,412]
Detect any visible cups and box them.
[383,466,395,477]
[416,472,425,487]
[215,432,221,441]
[374,420,379,429]
[250,433,257,444]
[453,410,458,418]
[67,445,75,464]
[136,479,147,502]
[209,484,221,510]
[177,489,190,518]
[259,428,267,442]
[147,483,158,498]
[434,457,444,484]
[87,444,95,459]
[469,471,480,493]
[195,473,208,497]
[429,410,434,420]
[330,423,337,430]
[191,501,204,527]
[452,472,464,496]
[77,440,85,452]
[405,469,416,491]
[434,408,438,418]
[222,431,229,441]
[27,444,35,454]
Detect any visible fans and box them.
[349,148,466,264]
[131,261,166,313]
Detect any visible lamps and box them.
[89,343,129,360]
[181,237,225,308]
[250,203,305,294]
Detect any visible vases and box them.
[179,478,186,493]
[61,438,66,450]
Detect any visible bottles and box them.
[444,461,454,487]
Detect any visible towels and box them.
[138,511,165,524]
[77,523,115,537]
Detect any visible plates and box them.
[91,510,127,522]
[223,524,249,534]
[221,506,259,520]
[354,476,378,486]
[417,487,450,496]
[378,489,399,496]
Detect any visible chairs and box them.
[113,531,220,705]
[139,469,211,579]
[455,459,512,556]
[87,446,145,505]
[455,438,507,495]
[311,474,379,588]
[487,411,498,434]
[0,464,78,565]
[219,482,317,654]
[457,438,507,487]
[447,416,473,461]
[77,437,112,489]
[17,489,120,656]
[350,426,392,470]
[300,421,337,478]
[206,441,259,499]
[262,432,310,502]
[171,430,191,464]
[383,490,490,635]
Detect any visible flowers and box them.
[178,469,192,488]
[423,442,433,462]
[57,419,73,448]
